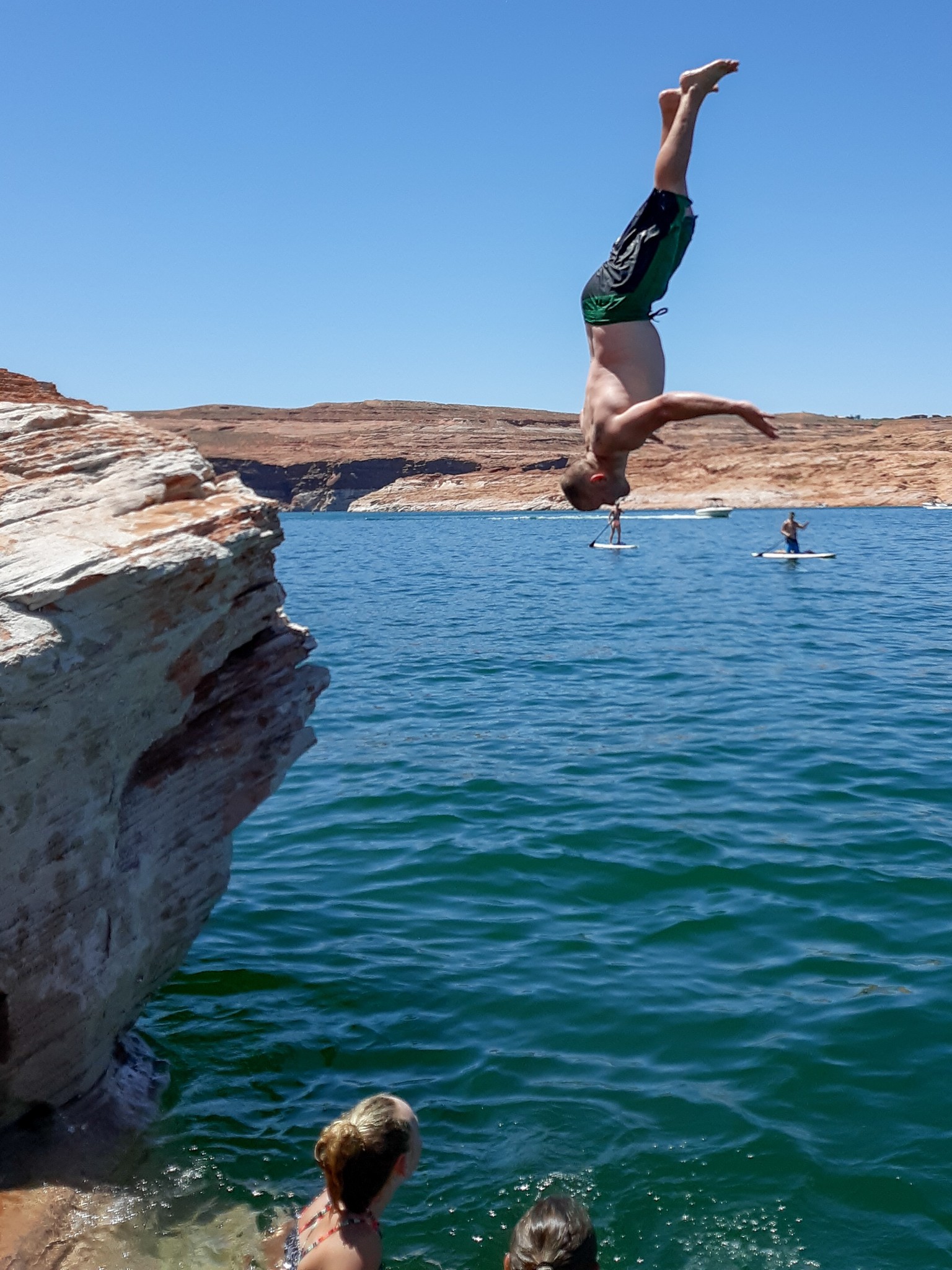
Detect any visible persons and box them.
[284,1093,422,1270]
[558,58,782,512]
[780,511,810,553]
[503,1196,600,1270]
[608,501,625,545]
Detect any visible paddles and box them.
[754,521,809,557]
[589,509,623,547]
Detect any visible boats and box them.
[695,497,733,517]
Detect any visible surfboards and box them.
[589,543,638,549]
[751,551,836,558]
